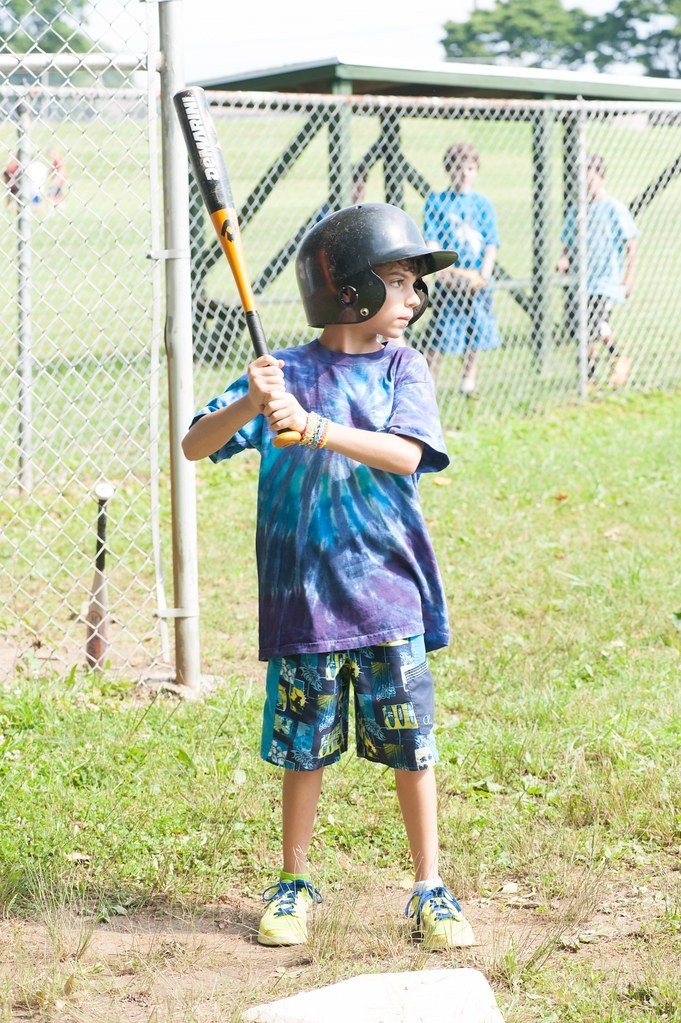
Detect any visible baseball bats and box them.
[174,85,270,357]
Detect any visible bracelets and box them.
[298,411,331,449]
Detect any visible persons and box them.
[181,202,475,948]
[423,143,500,393]
[555,153,638,392]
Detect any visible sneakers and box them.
[257,871,323,945]
[404,877,476,950]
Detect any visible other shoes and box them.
[574,357,595,381]
[458,378,481,400]
[608,344,620,364]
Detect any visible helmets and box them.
[295,203,458,328]
